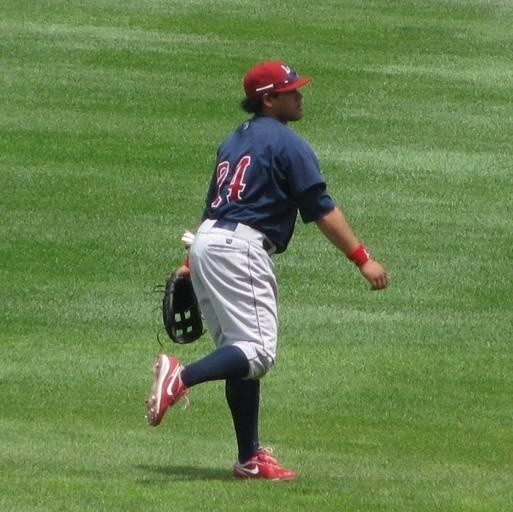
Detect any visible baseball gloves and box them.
[150,265,203,345]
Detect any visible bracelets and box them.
[347,241,371,267]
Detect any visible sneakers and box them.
[233,446,296,481]
[144,354,190,427]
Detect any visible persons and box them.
[145,58,389,480]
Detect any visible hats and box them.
[244,62,312,99]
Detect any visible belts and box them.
[213,219,272,251]
